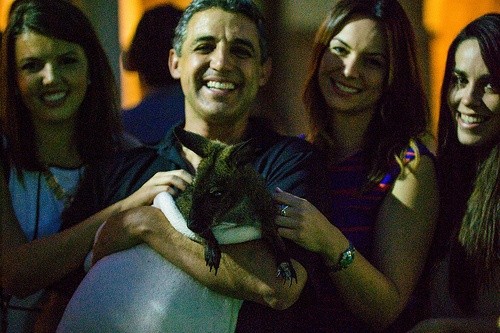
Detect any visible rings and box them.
[280,205,289,216]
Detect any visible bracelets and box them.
[325,240,356,273]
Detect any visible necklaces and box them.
[38,156,73,209]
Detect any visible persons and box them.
[0,0,196,333]
[118,2,185,146]
[397,12,500,333]
[27,0,320,333]
[271,0,436,332]
